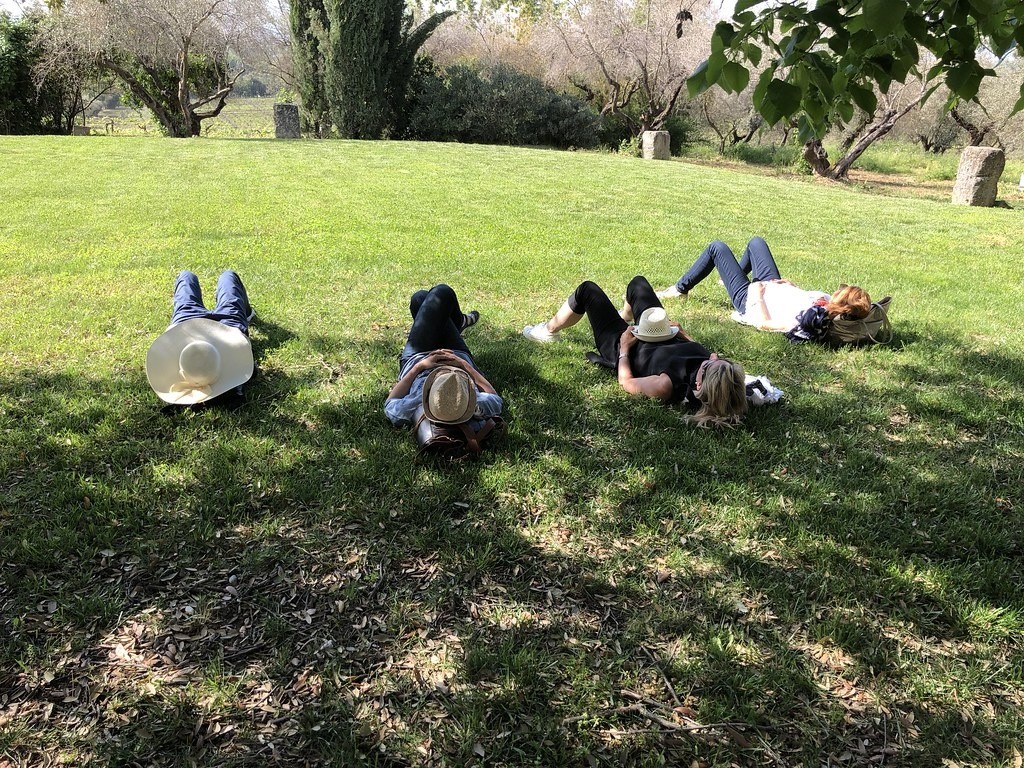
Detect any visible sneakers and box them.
[618,309,633,324]
[524,323,560,345]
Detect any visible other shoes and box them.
[459,311,479,333]
[654,286,688,299]
[719,280,724,285]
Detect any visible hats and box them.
[146,317,254,405]
[630,306,679,342]
[422,365,476,425]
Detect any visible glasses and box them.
[839,284,848,290]
[701,359,733,384]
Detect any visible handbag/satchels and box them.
[411,402,508,463]
[829,297,893,349]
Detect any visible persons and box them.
[523,275,746,430]
[383,284,504,424]
[144,270,256,404]
[657,235,872,339]
